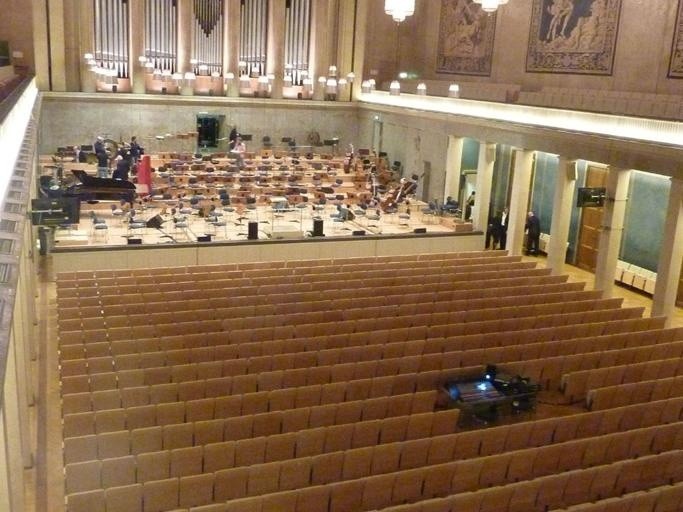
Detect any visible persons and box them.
[72,145,86,163]
[337,205,356,220]
[486,206,508,250]
[465,191,475,219]
[228,124,240,149]
[230,137,245,165]
[446,196,458,205]
[525,212,540,257]
[94,136,144,181]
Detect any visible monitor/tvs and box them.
[31,197,81,226]
[576,187,605,206]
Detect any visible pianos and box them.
[64,169,136,208]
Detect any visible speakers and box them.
[197,236,211,241]
[146,215,165,228]
[313,217,323,235]
[339,208,355,219]
[487,143,496,163]
[352,231,366,235]
[414,228,426,232]
[128,239,142,245]
[247,222,259,239]
[567,160,576,181]
